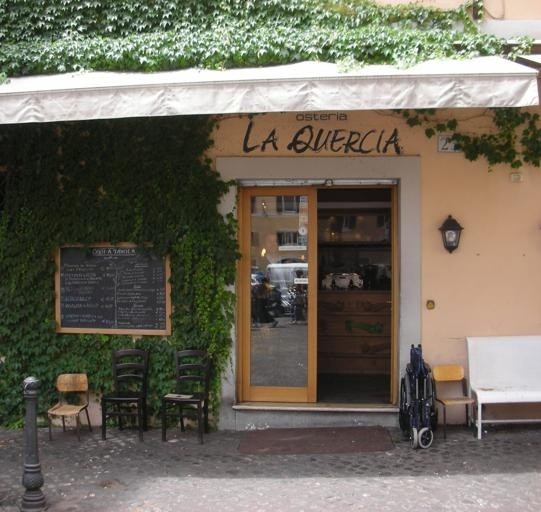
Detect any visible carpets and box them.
[236,424,400,457]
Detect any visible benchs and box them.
[464,335,541,441]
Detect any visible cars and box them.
[252,263,310,314]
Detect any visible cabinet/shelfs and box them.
[318,206,392,286]
[317,286,392,378]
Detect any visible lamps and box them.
[437,213,465,254]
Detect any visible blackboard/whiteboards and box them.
[54,241,172,336]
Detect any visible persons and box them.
[252,276,278,328]
[288,270,307,325]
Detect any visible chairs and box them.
[45,372,94,444]
[158,347,216,447]
[429,364,476,443]
[99,347,151,445]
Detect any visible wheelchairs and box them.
[400,343,440,450]
